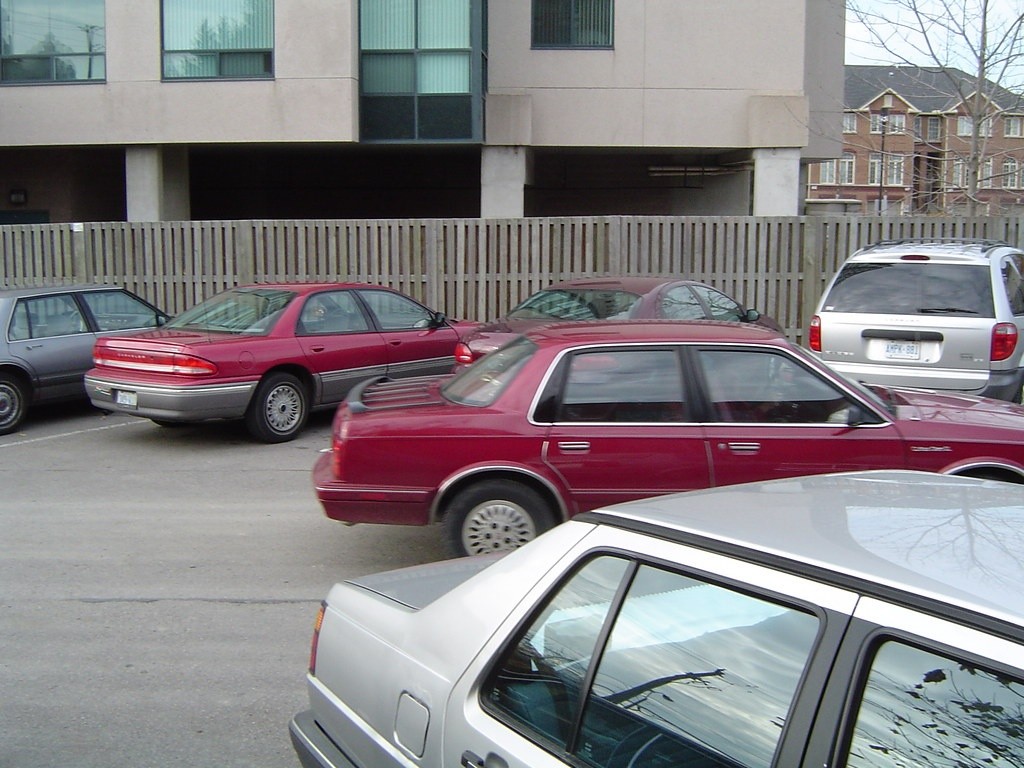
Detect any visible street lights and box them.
[876,106,891,217]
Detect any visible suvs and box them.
[810,235,1023,403]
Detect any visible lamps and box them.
[10,188,28,204]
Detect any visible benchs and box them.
[504,614,813,768]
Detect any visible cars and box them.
[0,286,179,436]
[83,280,484,445]
[308,315,1024,560]
[449,276,791,382]
[289,467,1024,768]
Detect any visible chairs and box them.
[705,369,757,422]
[13,312,39,339]
[46,311,83,334]
